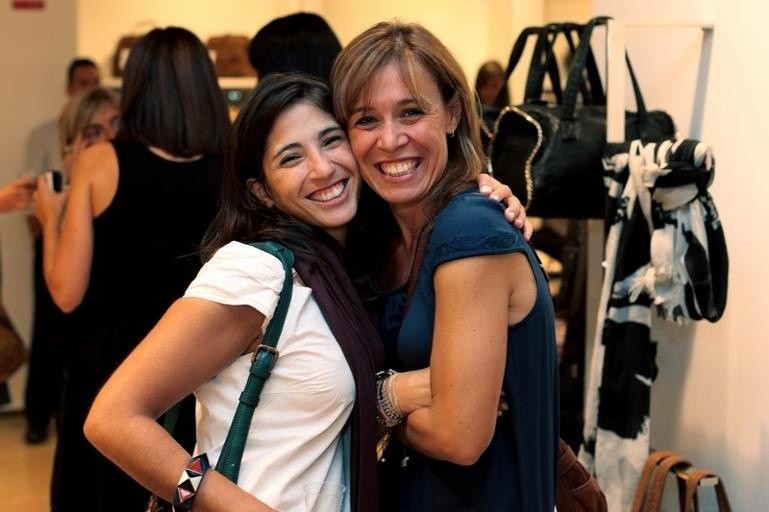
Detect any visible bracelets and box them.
[371,366,406,430]
[169,450,211,510]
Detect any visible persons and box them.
[55,84,125,185]
[1,176,38,217]
[30,24,237,510]
[80,69,538,510]
[247,10,344,84]
[20,57,105,446]
[330,21,560,512]
[472,59,508,156]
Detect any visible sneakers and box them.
[25,414,48,442]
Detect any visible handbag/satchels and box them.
[202,35,259,77]
[484,15,678,220]
[477,21,567,158]
[528,245,574,311]
[111,34,141,78]
[555,436,609,512]
